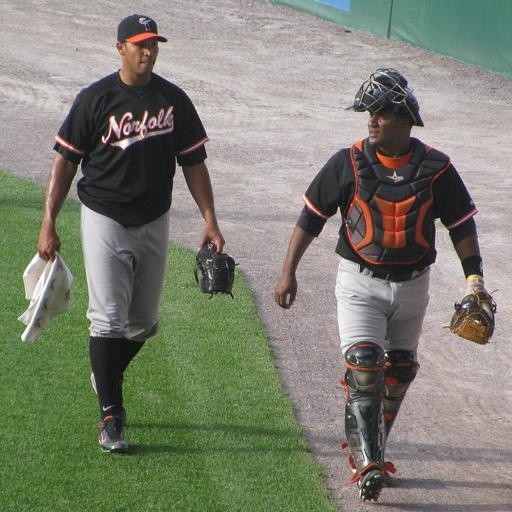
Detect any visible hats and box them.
[118,14,167,43]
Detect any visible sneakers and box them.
[357,469,383,501]
[90,371,128,453]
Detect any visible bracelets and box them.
[461,255,483,278]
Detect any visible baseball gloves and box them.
[195,242,240,300]
[451,286,494,345]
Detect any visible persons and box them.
[274,69,497,501]
[39,13,225,454]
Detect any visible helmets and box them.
[345,67,424,127]
